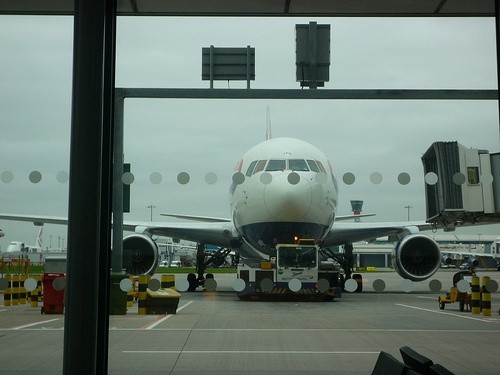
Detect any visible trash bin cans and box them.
[40,273,66,314]
[110,272,130,315]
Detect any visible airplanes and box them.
[0,137,500,299]
